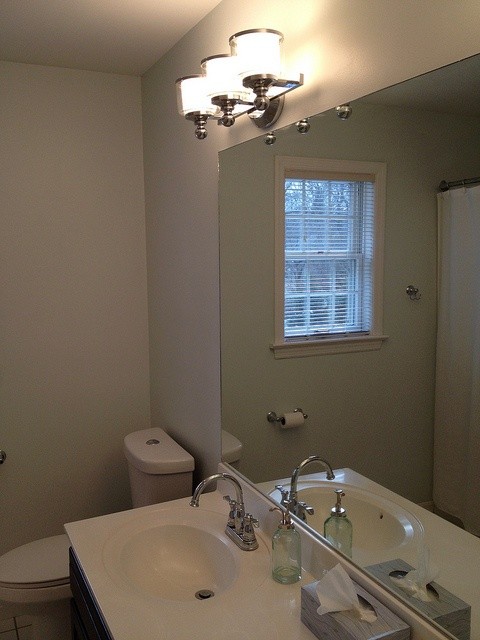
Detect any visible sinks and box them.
[119,524,240,606]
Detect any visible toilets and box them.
[1,427,195,639]
[222,427,243,472]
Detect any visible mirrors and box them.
[219,53,480,640]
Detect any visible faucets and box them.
[190,473,260,552]
[275,455,335,523]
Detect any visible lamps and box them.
[229,27,303,111]
[200,55,258,128]
[175,74,236,139]
